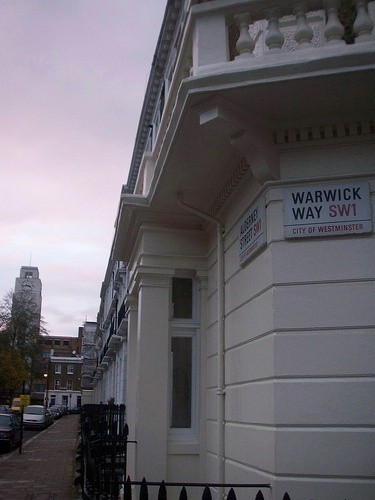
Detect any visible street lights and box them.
[44,374,49,407]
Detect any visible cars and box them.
[48,404,81,420]
[0,404,13,413]
[0,413,24,451]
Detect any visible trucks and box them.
[11,395,32,414]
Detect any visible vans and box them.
[21,406,50,428]
